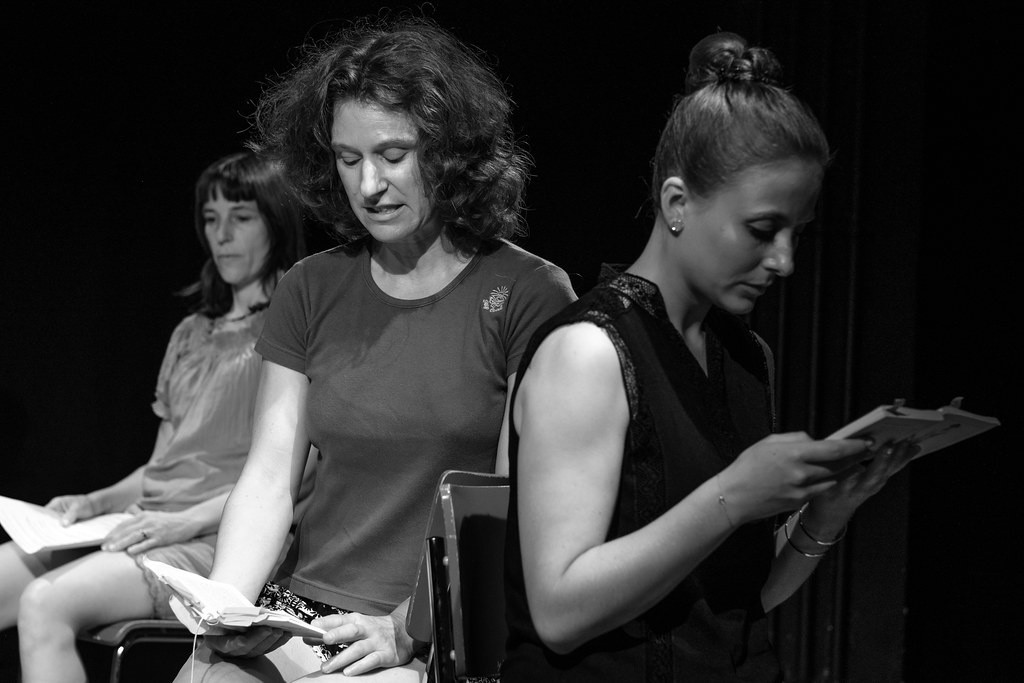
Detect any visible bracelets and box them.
[785,516,826,557]
[716,473,736,530]
[798,510,848,545]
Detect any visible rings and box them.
[140,528,148,539]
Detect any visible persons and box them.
[1,140,350,683]
[170,17,578,683]
[494,33,926,680]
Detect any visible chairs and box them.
[2,565,196,683]
[423,478,585,683]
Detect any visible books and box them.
[140,556,329,683]
[816,395,1002,472]
